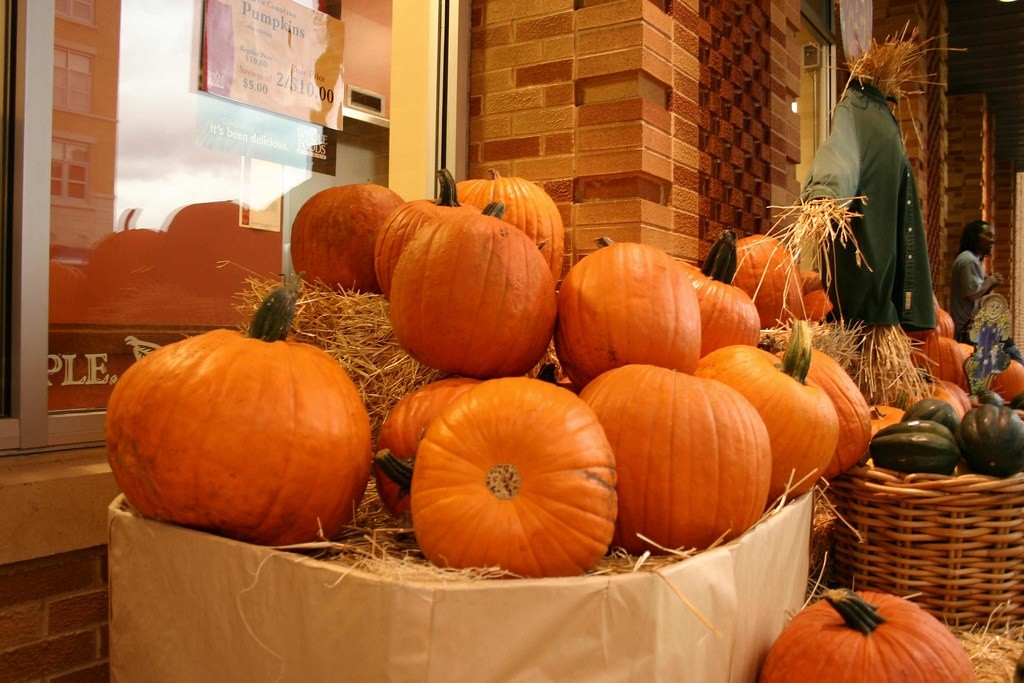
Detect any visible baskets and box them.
[823,459,1023,640]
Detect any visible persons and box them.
[950,220,1003,345]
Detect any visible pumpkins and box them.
[757,588,979,683]
[103,274,372,547]
[289,168,1024,580]
